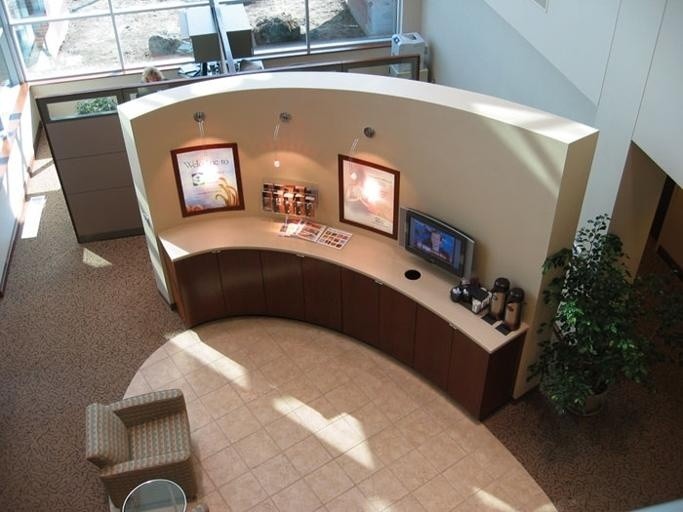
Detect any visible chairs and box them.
[84,389,199,510]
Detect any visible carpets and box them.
[108,318,557,512]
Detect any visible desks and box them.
[121,478,187,512]
[177,60,264,87]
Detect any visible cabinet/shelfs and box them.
[413,299,519,422]
[175,252,264,330]
[343,264,415,367]
[261,246,343,331]
[186,3,254,60]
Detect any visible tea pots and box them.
[490,278,525,330]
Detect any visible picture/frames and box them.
[337,153,400,241]
[169,143,246,218]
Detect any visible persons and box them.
[138,66,169,83]
[422,231,450,262]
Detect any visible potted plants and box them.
[522,212,683,429]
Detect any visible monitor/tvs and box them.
[398,207,475,283]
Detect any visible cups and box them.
[472,286,492,314]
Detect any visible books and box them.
[278,215,353,250]
